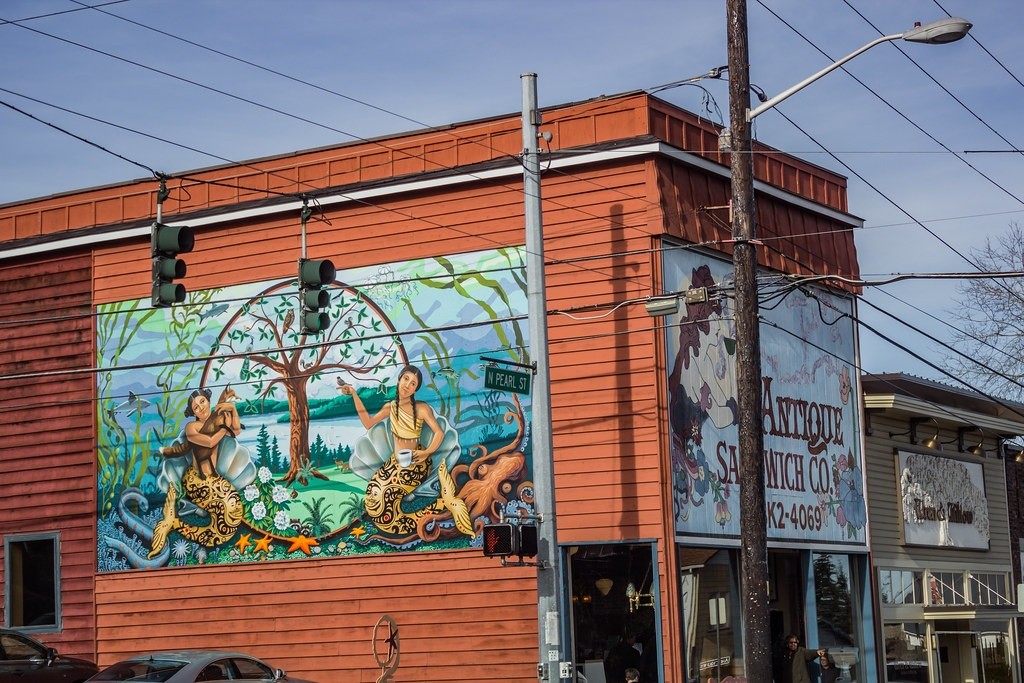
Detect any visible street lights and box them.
[728,17,972,683]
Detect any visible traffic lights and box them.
[147,221,195,312]
[514,524,540,559]
[479,522,520,559]
[295,256,337,335]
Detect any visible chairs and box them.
[205,665,223,681]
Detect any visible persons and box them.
[624,668,640,683]
[781,634,826,683]
[820,655,844,683]
[605,631,641,683]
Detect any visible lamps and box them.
[889,417,1024,464]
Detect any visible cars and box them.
[0,625,100,683]
[80,650,318,683]
